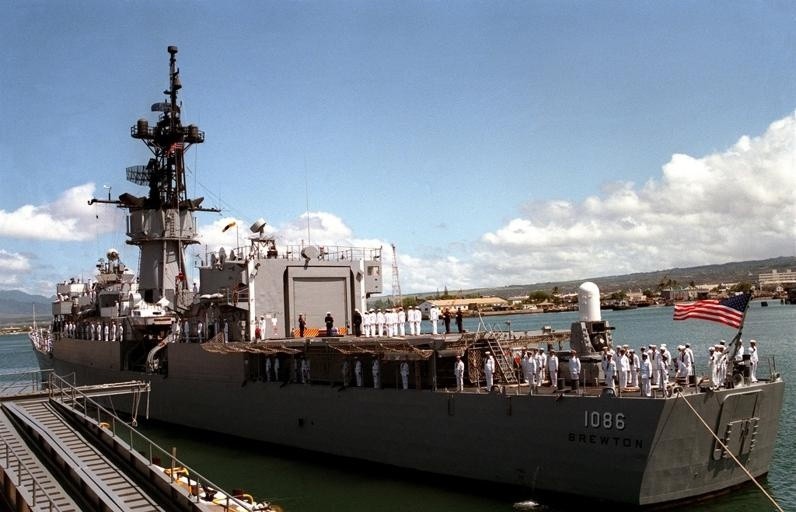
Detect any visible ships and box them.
[28,44,787,510]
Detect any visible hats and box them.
[484,351,490,355]
[519,347,578,357]
[677,343,690,351]
[708,340,756,352]
[602,344,666,357]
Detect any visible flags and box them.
[673,292,751,329]
[171,142,184,149]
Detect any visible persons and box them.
[454,356,464,391]
[483,352,495,391]
[30,321,123,353]
[299,313,306,337]
[265,356,312,385]
[602,342,695,396]
[342,355,410,390]
[256,316,266,342]
[569,350,582,390]
[707,338,758,390]
[351,307,422,338]
[325,313,333,336]
[172,318,228,344]
[428,306,463,335]
[514,347,559,394]
